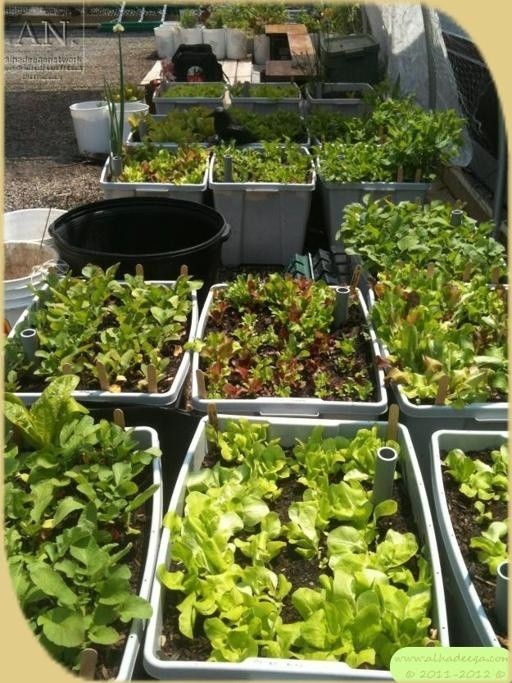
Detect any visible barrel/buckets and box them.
[153,22,271,65]
[108,102,150,142]
[3,208,66,246]
[49,196,232,298]
[68,101,111,155]
[2,243,58,322]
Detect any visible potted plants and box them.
[3,3,512,678]
